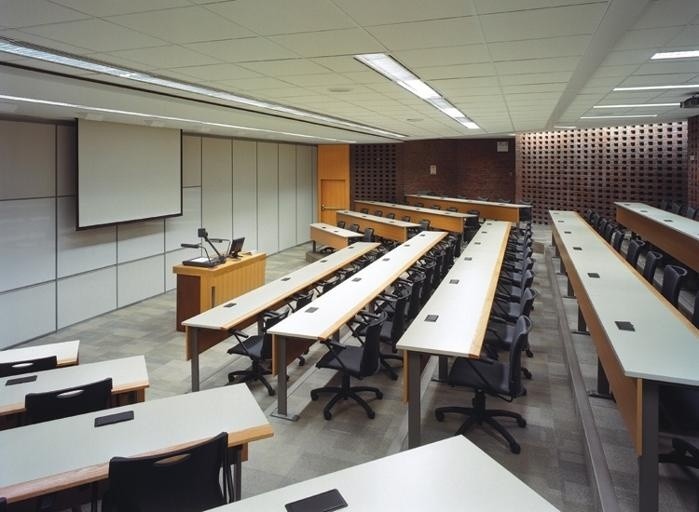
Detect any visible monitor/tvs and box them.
[229,237,245,258]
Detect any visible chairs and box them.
[506,238,533,252]
[336,220,347,228]
[428,191,436,195]
[102,432,235,512]
[419,218,431,230]
[424,242,454,279]
[508,231,532,244]
[398,201,407,205]
[336,269,357,283]
[401,215,411,221]
[446,207,458,212]
[439,193,449,196]
[509,225,531,235]
[430,205,441,209]
[660,264,688,309]
[605,223,615,244]
[310,312,389,420]
[353,284,413,380]
[691,293,698,328]
[386,213,395,219]
[1,355,58,377]
[349,223,360,233]
[466,210,481,228]
[504,247,533,261]
[660,200,668,210]
[518,199,533,217]
[373,210,383,216]
[382,267,426,322]
[592,212,600,230]
[351,259,372,272]
[362,226,374,243]
[433,315,529,455]
[482,285,534,396]
[600,218,608,237]
[686,206,697,220]
[456,195,468,199]
[226,308,291,396]
[626,237,646,269]
[643,250,664,284]
[495,268,534,302]
[411,254,437,301]
[270,292,315,366]
[671,202,682,214]
[613,230,625,253]
[496,198,512,204]
[356,251,377,263]
[659,434,698,469]
[24,378,114,426]
[586,208,592,223]
[500,256,534,285]
[446,234,457,253]
[314,279,341,297]
[414,202,424,207]
[359,207,369,213]
[477,196,490,201]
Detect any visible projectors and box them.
[181,225,226,266]
[680,92,699,109]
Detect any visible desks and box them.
[180,241,383,392]
[0,384,274,512]
[267,229,449,422]
[203,434,562,512]
[614,199,699,273]
[310,221,364,254]
[548,209,699,512]
[1,354,149,432]
[173,251,266,331]
[354,198,479,235]
[335,210,423,247]
[407,193,533,226]
[0,339,80,367]
[396,219,514,449]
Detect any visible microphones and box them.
[181,243,199,249]
